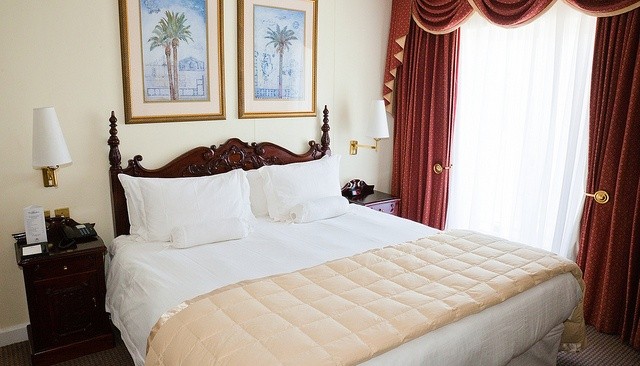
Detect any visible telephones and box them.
[57,221,99,250]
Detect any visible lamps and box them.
[31,107,72,187]
[351,100,390,155]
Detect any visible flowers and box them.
[237,1,318,117]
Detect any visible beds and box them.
[102,105,588,365]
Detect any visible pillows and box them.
[290,196,349,222]
[117,168,256,240]
[170,220,245,247]
[258,155,349,222]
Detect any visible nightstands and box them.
[11,227,115,365]
[341,179,401,216]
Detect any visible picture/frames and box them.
[118,0,227,124]
